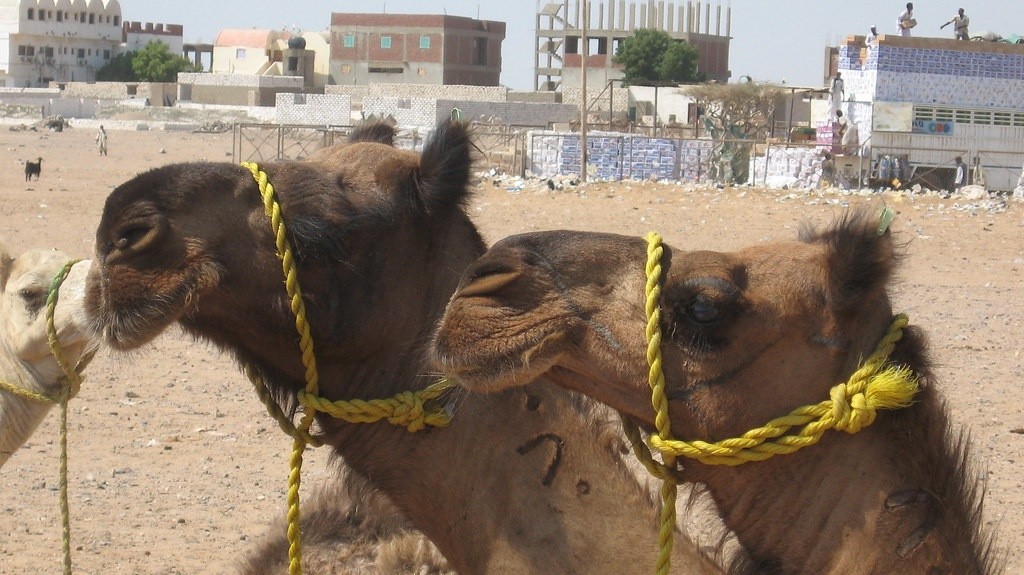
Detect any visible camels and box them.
[1,238,101,469]
[85,115,993,575]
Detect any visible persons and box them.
[825,72,845,120]
[896,3,914,36]
[865,25,880,51]
[837,110,856,156]
[96,126,107,155]
[940,8,969,40]
[972,157,986,185]
[955,156,967,188]
